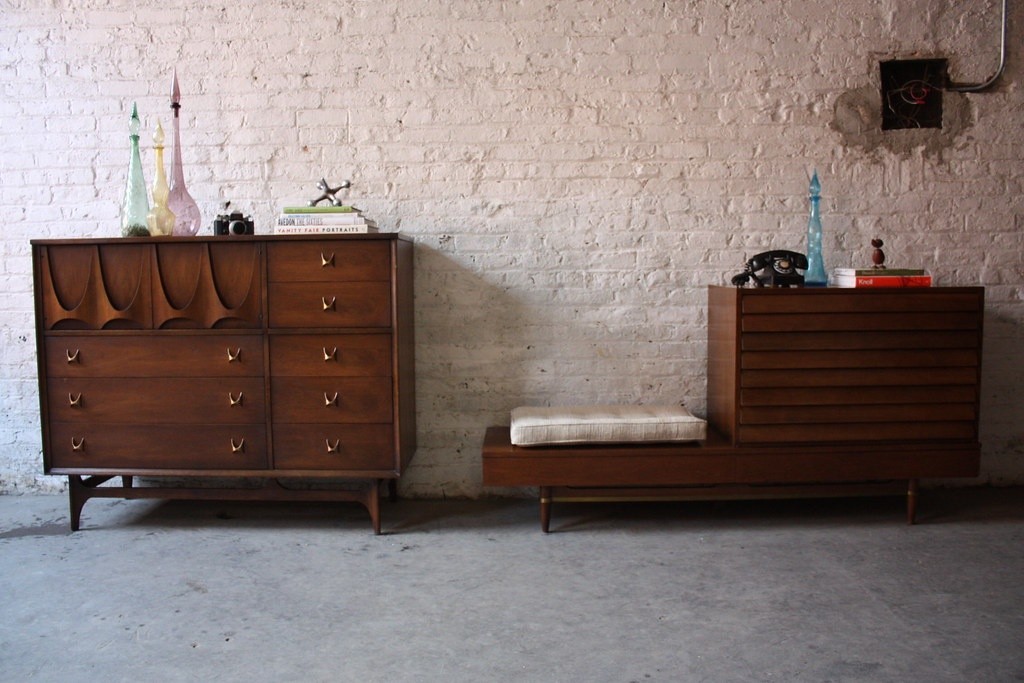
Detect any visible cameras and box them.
[213,211,254,235]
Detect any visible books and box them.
[828,267,932,288]
[273,205,379,235]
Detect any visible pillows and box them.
[510,403,706,448]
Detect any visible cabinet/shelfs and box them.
[482,285,984,533]
[30,232,417,535]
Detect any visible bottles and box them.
[121,66,201,236]
[808,168,826,285]
